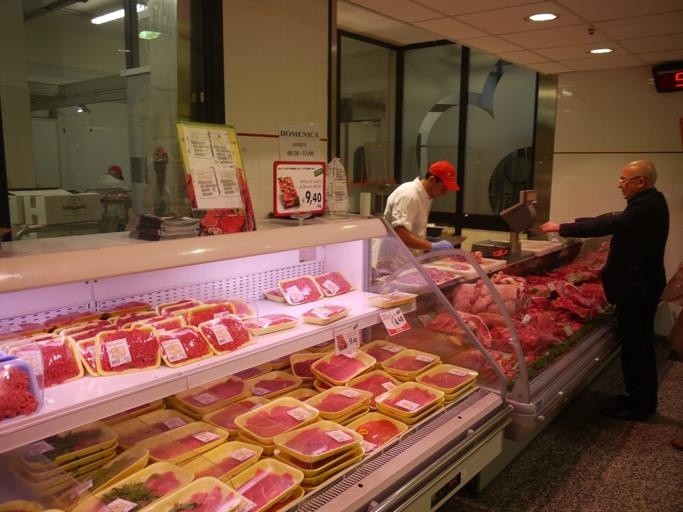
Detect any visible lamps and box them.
[90,2,148,26]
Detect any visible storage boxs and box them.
[7,188,101,240]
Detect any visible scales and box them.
[471,190,538,260]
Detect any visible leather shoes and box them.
[600,394,655,422]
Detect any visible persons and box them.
[374,160,461,273]
[523,158,670,420]
[144,146,179,218]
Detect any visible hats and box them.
[428,161,461,192]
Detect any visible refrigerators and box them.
[416,230,615,445]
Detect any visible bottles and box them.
[360,193,371,217]
